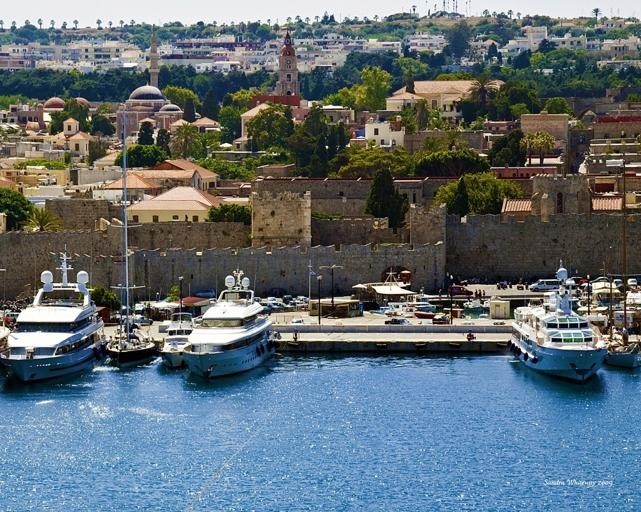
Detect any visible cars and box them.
[432,314,449,324]
[260,294,309,316]
[529,274,638,315]
[290,318,304,326]
[448,285,473,296]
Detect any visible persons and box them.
[475,289,485,297]
[439,289,442,300]
[525,280,528,290]
[420,286,425,296]
[109,336,115,349]
[497,281,500,289]
[622,326,629,345]
[508,280,512,288]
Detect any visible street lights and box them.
[450,275,454,324]
[308,259,344,324]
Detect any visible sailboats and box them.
[606,160,641,367]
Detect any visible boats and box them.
[0,245,277,380]
[510,259,607,381]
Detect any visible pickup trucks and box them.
[385,317,412,325]
